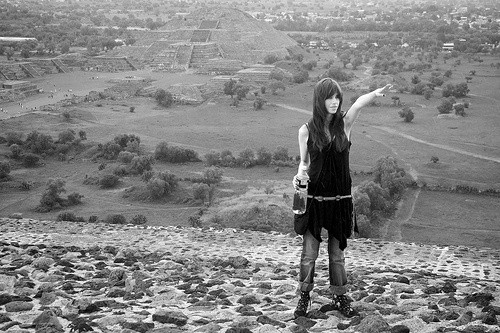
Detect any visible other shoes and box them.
[295,292,309,318]
[331,294,356,317]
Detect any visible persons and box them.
[293,78,397,318]
